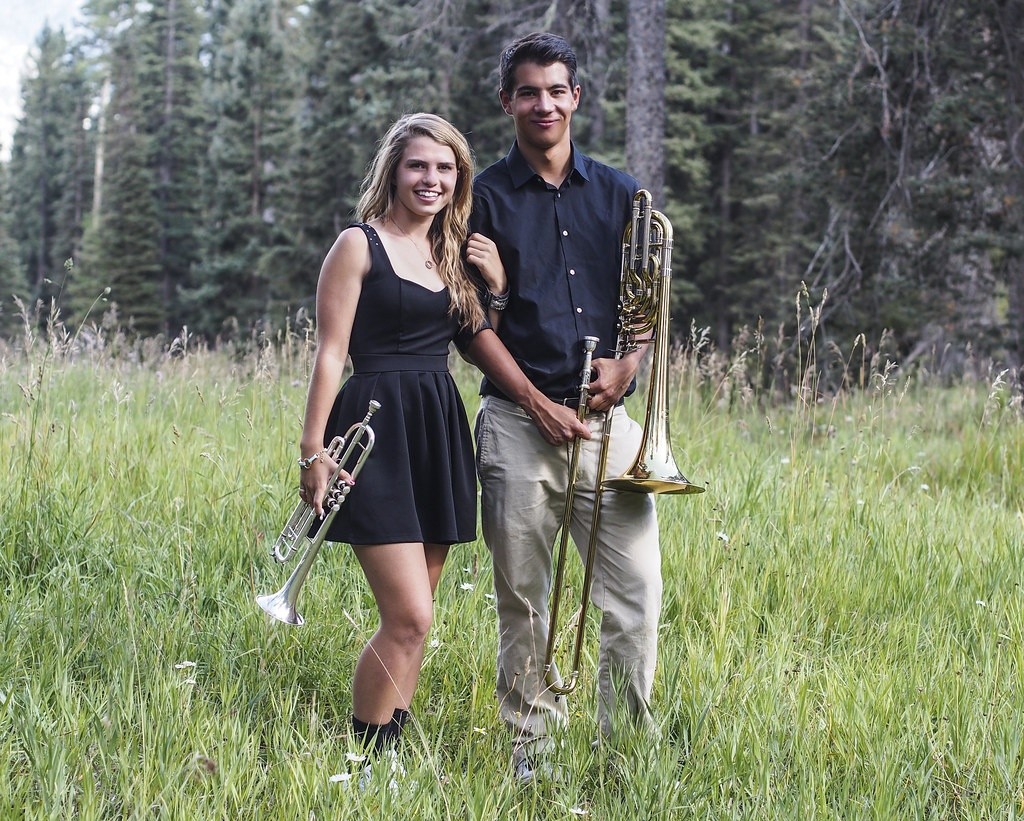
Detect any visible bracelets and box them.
[298,447,328,470]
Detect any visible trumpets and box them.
[254,400,382,626]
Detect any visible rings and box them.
[299,487,305,493]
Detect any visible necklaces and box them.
[384,211,434,269]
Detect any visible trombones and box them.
[542,190,707,703]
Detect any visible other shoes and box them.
[344,750,420,805]
[517,753,576,786]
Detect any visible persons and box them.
[458,32,670,800]
[296,113,511,796]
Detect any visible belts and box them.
[481,378,624,415]
